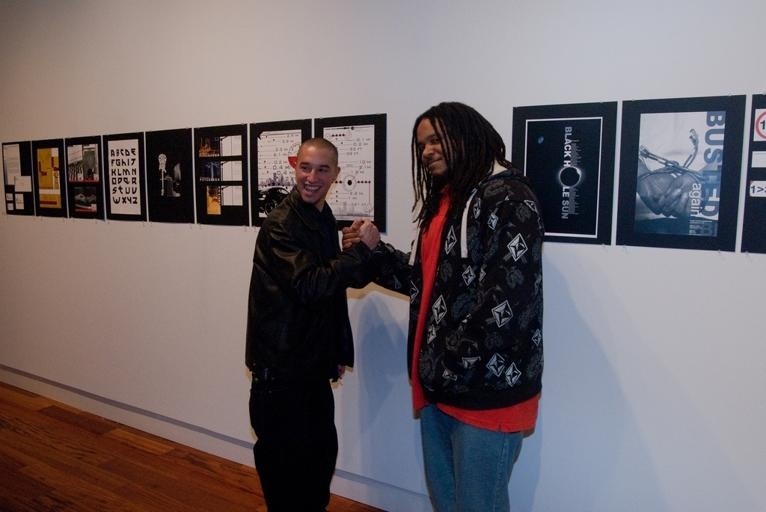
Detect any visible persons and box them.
[342,103,548,512]
[636,153,723,219]
[245,139,381,511]
